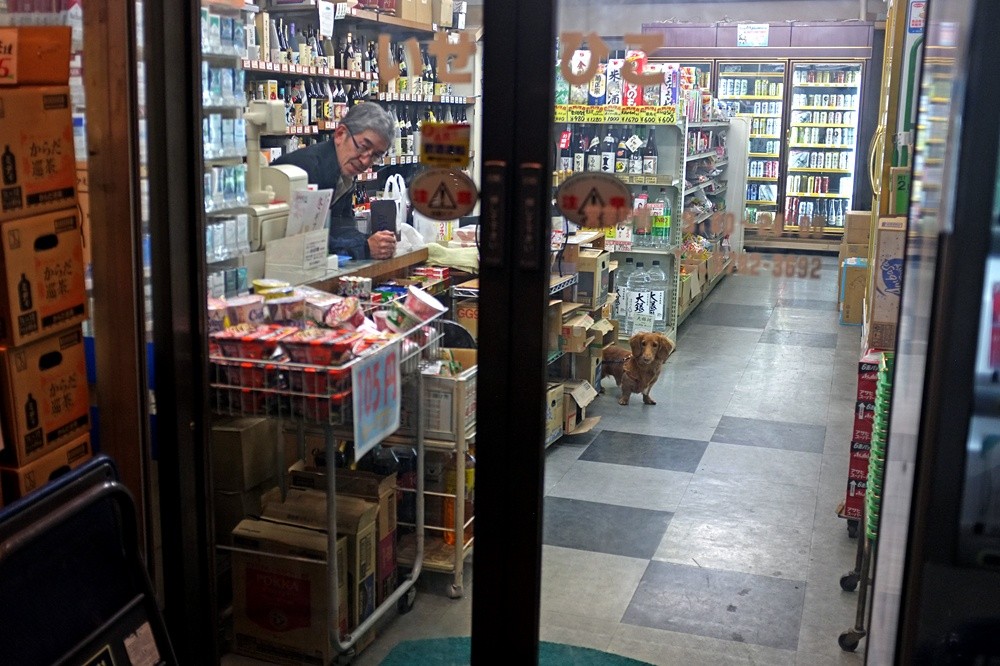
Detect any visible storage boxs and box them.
[1,24,91,499]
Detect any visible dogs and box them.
[596,333,675,407]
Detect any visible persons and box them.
[267,101,397,262]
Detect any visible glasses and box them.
[345,122,384,166]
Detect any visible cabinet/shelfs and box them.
[201,0,999,666]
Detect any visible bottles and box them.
[255,8,470,218]
[315,434,476,546]
[634,186,652,247]
[624,262,652,335]
[815,199,849,229]
[553,122,658,176]
[552,191,648,255]
[645,260,667,332]
[651,187,671,251]
[613,257,636,335]
